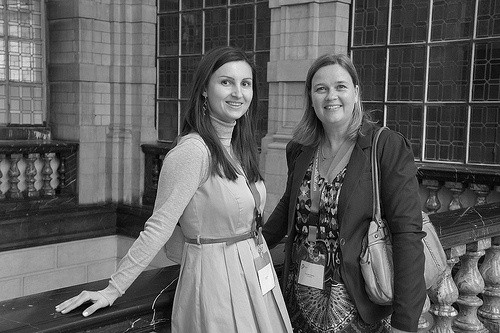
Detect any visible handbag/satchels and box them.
[357,209,448,305]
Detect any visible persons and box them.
[55,46,294,332]
[261,52,426,333]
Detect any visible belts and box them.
[185,230,257,246]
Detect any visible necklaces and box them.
[322,133,336,161]
